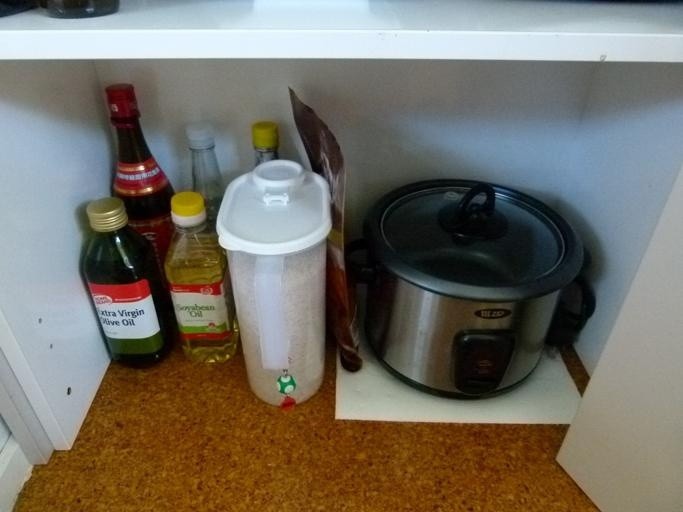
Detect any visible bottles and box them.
[84,196,172,368]
[163,191,239,366]
[182,118,225,198]
[97,82,175,252]
[251,120,279,169]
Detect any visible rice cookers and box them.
[348,177,597,402]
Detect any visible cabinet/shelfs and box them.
[2,0,682,512]
